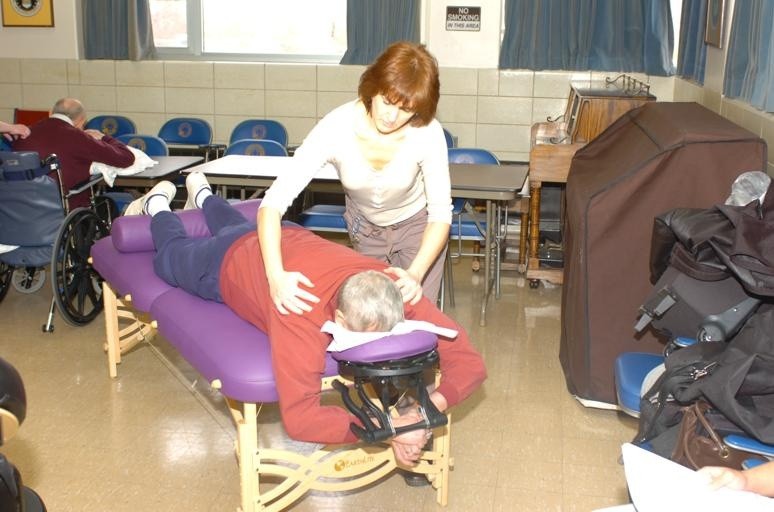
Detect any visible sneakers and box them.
[182,170,212,210]
[123,179,178,217]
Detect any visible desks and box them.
[180,154,531,326]
[98,156,204,189]
[528,75,655,290]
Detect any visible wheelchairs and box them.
[0,151,112,333]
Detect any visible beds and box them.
[87,201,452,511]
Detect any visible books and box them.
[620,442,774,511]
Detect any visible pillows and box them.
[112,198,263,251]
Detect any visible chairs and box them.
[84,116,136,139]
[227,119,288,145]
[300,198,351,236]
[158,118,215,205]
[215,140,287,157]
[442,131,453,148]
[614,336,772,458]
[448,149,507,299]
[114,135,168,198]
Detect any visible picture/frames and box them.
[1,1,53,27]
[704,0,725,52]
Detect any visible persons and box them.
[124,170,488,468]
[0,121,31,142]
[257,42,454,487]
[697,461,774,498]
[11,98,136,219]
